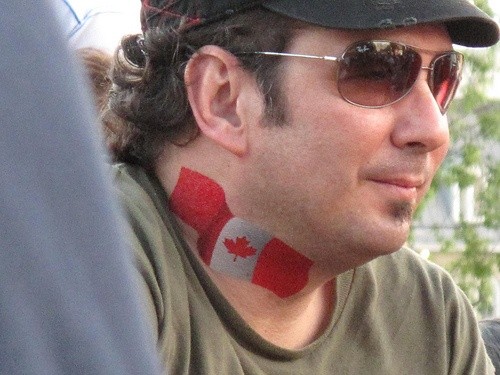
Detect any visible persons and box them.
[100,0,500,375]
[76,47,114,115]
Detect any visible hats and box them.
[140,0,500,48]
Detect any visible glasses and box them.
[232,39,465,116]
